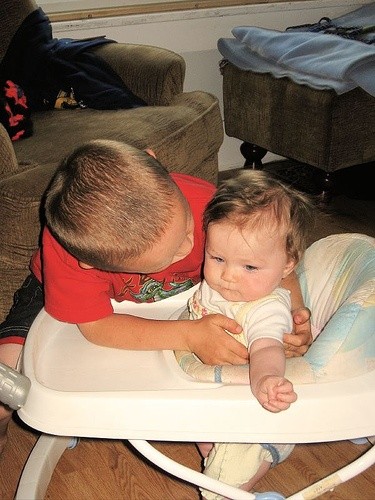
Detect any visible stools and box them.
[217,57,375,212]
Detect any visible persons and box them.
[187,172,314,500]
[0,140,314,459]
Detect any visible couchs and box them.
[0,0,224,324]
